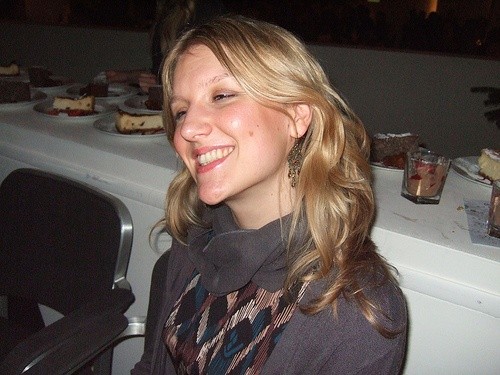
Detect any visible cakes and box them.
[49,95,100,117]
[0,80,32,104]
[31,67,65,87]
[0,62,20,77]
[114,111,163,133]
[139,73,159,93]
[144,86,164,110]
[478,149,500,181]
[371,132,422,167]
[80,71,110,97]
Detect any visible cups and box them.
[486,179,500,239]
[400,146,452,205]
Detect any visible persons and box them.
[106,0,196,93]
[256,0,500,56]
[130,14,408,375]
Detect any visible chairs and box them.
[0,167,134,375]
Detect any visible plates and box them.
[0,92,47,108]
[31,98,118,121]
[368,160,404,173]
[93,113,167,138]
[450,155,493,188]
[118,94,163,115]
[107,83,138,103]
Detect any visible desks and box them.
[0,103,500,375]
